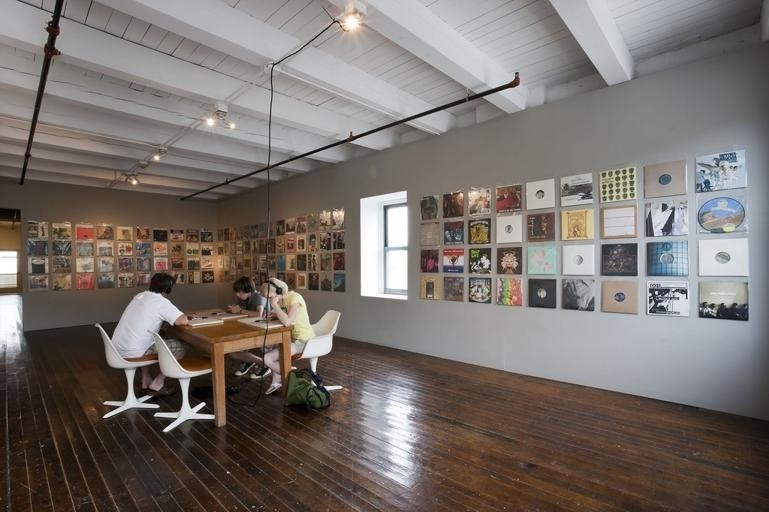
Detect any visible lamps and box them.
[155,145,168,161]
[206,103,236,129]
[124,172,140,186]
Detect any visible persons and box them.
[110,273,189,397]
[228,276,272,380]
[260,278,316,396]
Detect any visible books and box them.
[188,318,224,327]
[238,316,284,330]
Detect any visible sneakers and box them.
[250,365,272,379]
[234,360,256,376]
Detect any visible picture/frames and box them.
[600,205,638,239]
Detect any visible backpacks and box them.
[281,365,332,410]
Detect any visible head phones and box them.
[263,279,283,295]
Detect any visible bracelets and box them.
[240,308,242,314]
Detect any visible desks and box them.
[164,308,294,427]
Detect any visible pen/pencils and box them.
[254,320,268,322]
[211,312,223,314]
[195,317,208,319]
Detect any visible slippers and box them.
[146,382,177,396]
[139,387,150,394]
[264,383,283,396]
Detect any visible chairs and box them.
[291,310,343,391]
[147,330,215,432]
[95,323,161,418]
